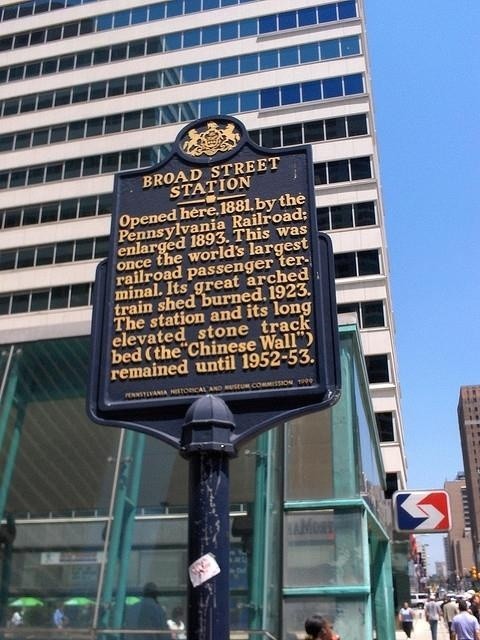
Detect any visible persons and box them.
[11,609,24,626]
[124,582,172,640]
[440,592,480,640]
[425,595,442,640]
[166,607,188,640]
[399,602,416,639]
[53,601,64,628]
[306,616,340,640]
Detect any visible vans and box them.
[410,593,429,609]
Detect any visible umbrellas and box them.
[8,597,45,608]
[64,597,95,605]
